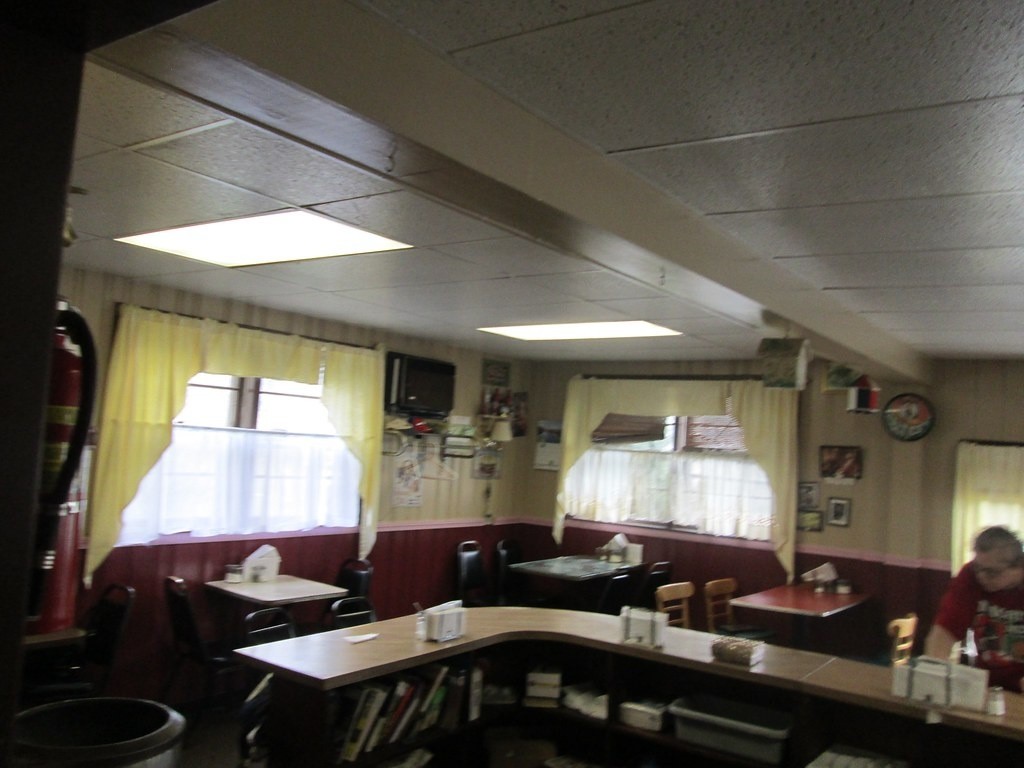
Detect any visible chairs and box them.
[34,582,137,698]
[243,608,299,645]
[457,539,497,607]
[507,553,649,612]
[552,569,633,614]
[317,558,376,623]
[165,575,241,727]
[885,612,920,668]
[704,577,778,644]
[495,540,525,604]
[645,559,675,603]
[655,581,696,629]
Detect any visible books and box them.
[332,663,484,768]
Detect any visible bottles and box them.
[414,613,425,642]
[986,687,1006,716]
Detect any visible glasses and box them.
[972,564,1016,580]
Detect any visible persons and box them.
[821,447,859,479]
[923,525,1024,697]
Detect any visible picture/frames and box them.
[826,497,852,526]
[818,446,865,480]
[797,509,824,531]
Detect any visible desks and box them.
[203,573,350,611]
[726,582,874,656]
[21,624,96,688]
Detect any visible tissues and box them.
[242,543,281,582]
[423,601,468,643]
[617,607,668,650]
[890,653,989,712]
